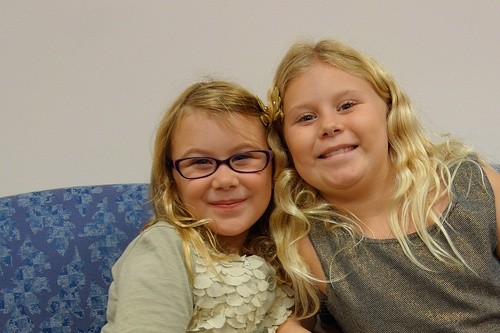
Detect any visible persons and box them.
[266,40,500,333]
[99,82,294,333]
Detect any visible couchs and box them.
[0,163,500,333]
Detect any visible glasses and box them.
[168,149,273,180]
